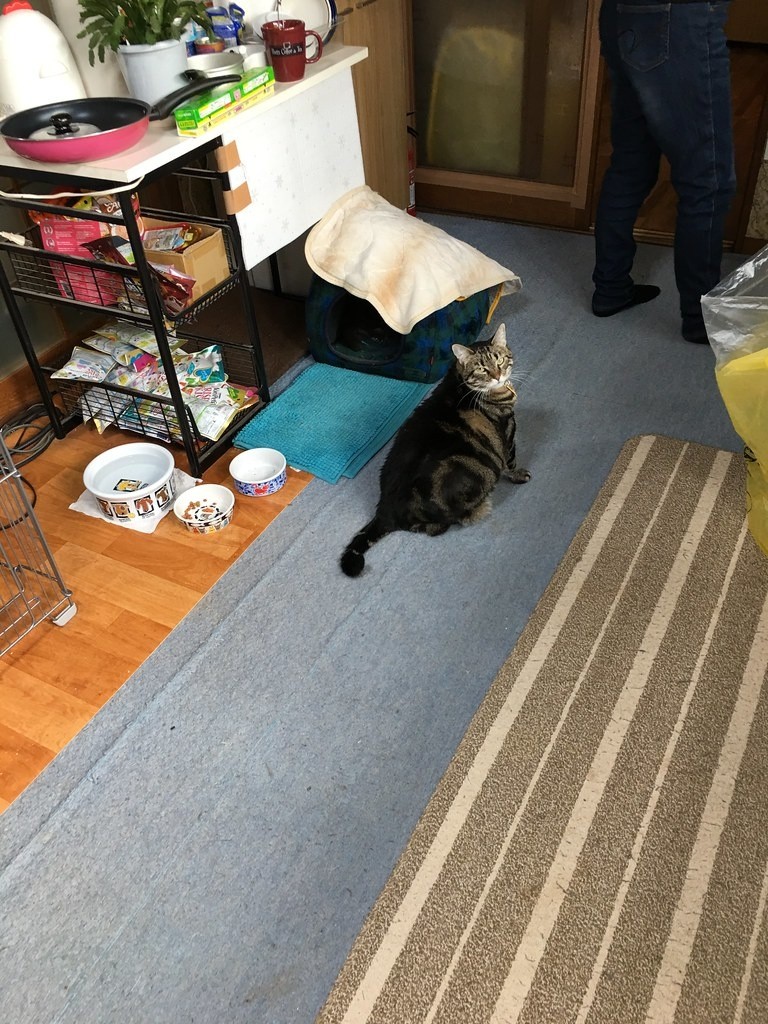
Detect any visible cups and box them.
[221,45,267,71]
[262,18,322,82]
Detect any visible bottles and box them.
[1,0,88,117]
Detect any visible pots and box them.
[1,72,243,164]
[183,54,245,88]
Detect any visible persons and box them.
[591,0,742,345]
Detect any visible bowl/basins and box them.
[173,483,235,535]
[229,448,288,495]
[86,442,174,523]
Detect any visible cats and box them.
[340,323,532,577]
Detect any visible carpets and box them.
[318,431,768,1024]
